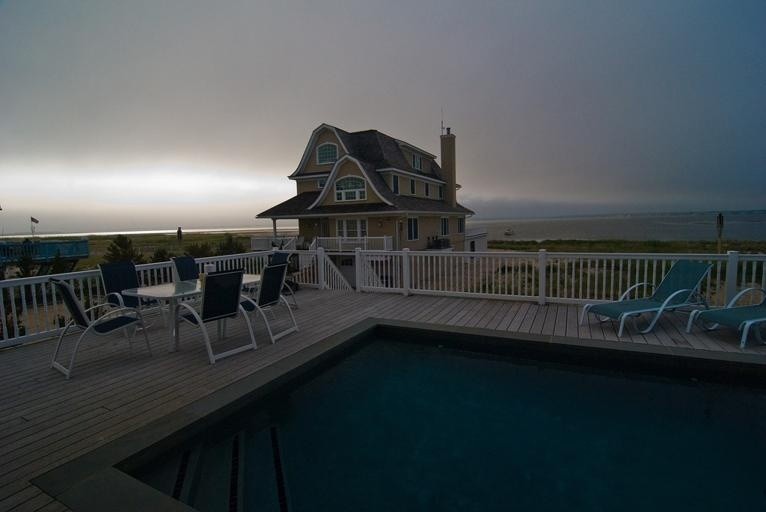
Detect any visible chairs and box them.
[222,260,301,345]
[572,256,719,338]
[171,252,204,318]
[94,255,169,343]
[173,267,260,367]
[685,281,766,352]
[248,250,299,311]
[42,273,153,383]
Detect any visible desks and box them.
[118,270,259,351]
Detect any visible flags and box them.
[32,217,39,224]
